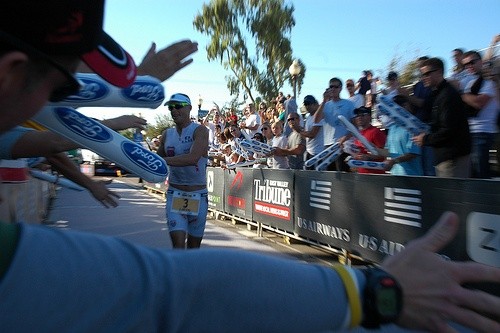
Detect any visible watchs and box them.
[357,264,404,328]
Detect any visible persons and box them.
[0,0,500,333]
[200,36,500,179]
[0,38,198,210]
[152,94,208,249]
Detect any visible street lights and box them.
[288,58,302,100]
[198,97,203,120]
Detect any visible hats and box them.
[0,1,137,90]
[303,95,316,104]
[353,106,370,114]
[156,135,162,139]
[386,72,398,79]
[164,94,191,106]
[152,138,158,143]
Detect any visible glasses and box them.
[419,69,441,79]
[462,57,481,68]
[288,117,298,123]
[261,128,268,131]
[37,56,83,103]
[387,78,396,81]
[168,103,189,111]
[304,103,313,106]
[330,84,341,89]
[253,136,261,139]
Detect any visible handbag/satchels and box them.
[458,92,483,119]
